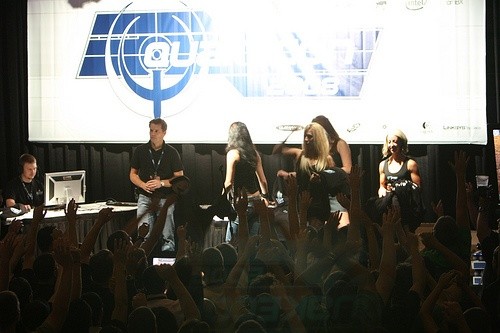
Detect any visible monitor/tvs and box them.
[43,171,86,206]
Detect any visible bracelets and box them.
[261,192,268,198]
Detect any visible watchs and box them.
[160,180,163,187]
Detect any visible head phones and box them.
[18,154,26,174]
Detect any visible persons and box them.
[312,115,353,175]
[0,149,500,333]
[379,129,422,198]
[221,121,270,245]
[272,122,336,225]
[130,119,185,258]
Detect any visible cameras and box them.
[471,184,495,204]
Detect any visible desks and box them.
[0,200,138,266]
